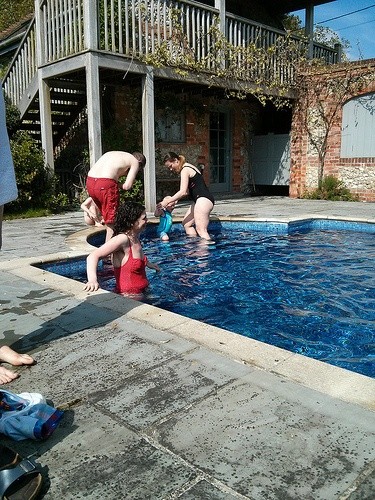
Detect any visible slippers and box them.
[0,444,42,500]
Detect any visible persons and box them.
[81,196,105,227]
[154,196,178,243]
[82,201,161,302]
[0,88,35,387]
[157,152,215,242]
[86,150,146,244]
